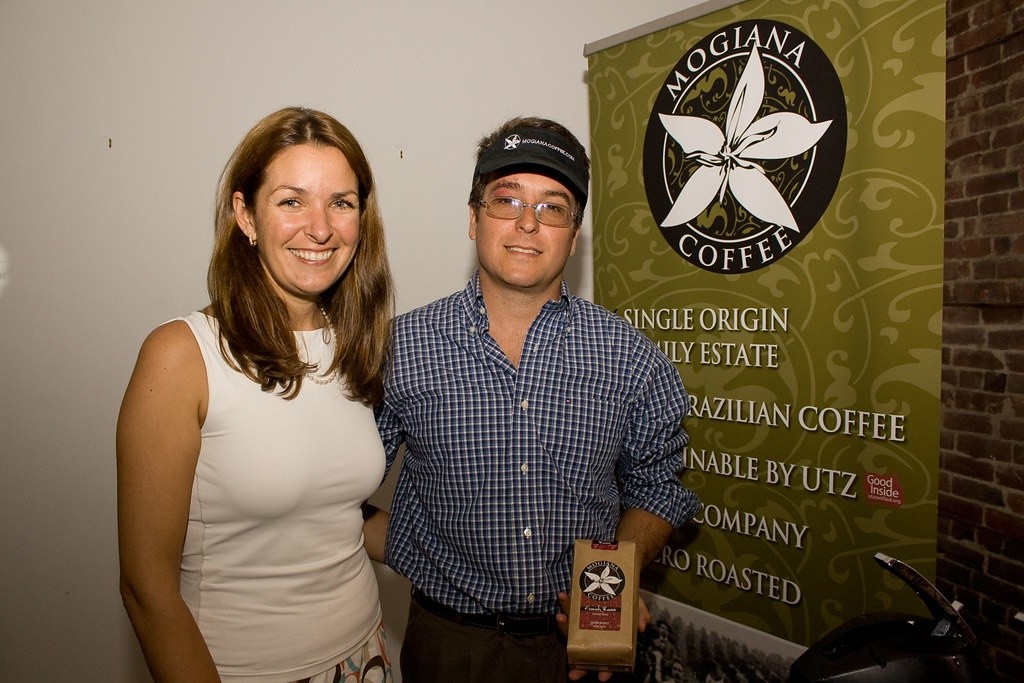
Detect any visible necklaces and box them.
[303,298,339,385]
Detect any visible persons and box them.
[373,117,701,683]
[116,107,394,683]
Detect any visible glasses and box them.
[477,197,579,228]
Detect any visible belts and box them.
[411,587,566,634]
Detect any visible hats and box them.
[475,129,589,208]
